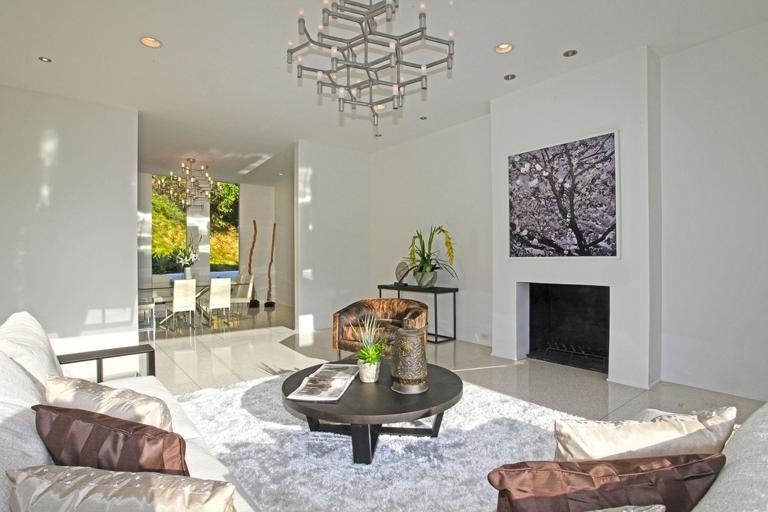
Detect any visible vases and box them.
[184,269,194,278]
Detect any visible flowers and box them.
[167,233,204,267]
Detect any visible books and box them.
[285,363,360,403]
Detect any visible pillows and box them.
[0,463,237,512]
[554,407,739,460]
[31,403,188,472]
[487,451,726,512]
[42,375,173,434]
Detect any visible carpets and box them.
[169,356,623,512]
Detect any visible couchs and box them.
[330,297,428,363]
[484,405,768,512]
[0,311,261,512]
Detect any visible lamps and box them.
[138,34,162,49]
[285,0,457,127]
[149,156,217,210]
[417,114,427,123]
[38,55,52,66]
[502,72,517,82]
[494,42,514,55]
[561,49,578,58]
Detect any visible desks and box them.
[377,284,459,344]
[159,280,249,329]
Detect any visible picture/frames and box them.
[507,132,620,259]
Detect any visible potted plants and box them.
[346,310,388,383]
[397,224,460,288]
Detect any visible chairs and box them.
[231,274,255,321]
[151,276,180,324]
[202,277,233,332]
[164,277,199,333]
